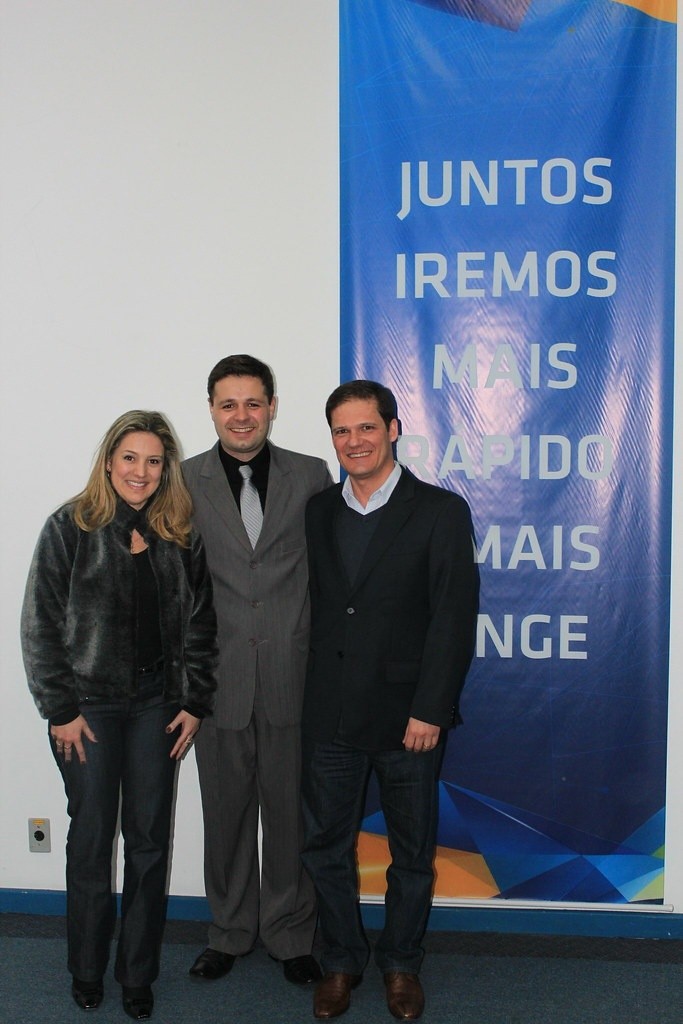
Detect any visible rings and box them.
[424,745,431,748]
[56,741,63,748]
[64,746,72,749]
[185,736,192,743]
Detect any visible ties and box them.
[238,464,264,550]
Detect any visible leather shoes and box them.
[188,947,237,981]
[268,953,321,984]
[123,984,154,1021]
[384,971,424,1022]
[71,977,105,1010]
[313,972,364,1020]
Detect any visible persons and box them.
[179,354,335,985]
[303,380,472,1020]
[22,410,217,1020]
